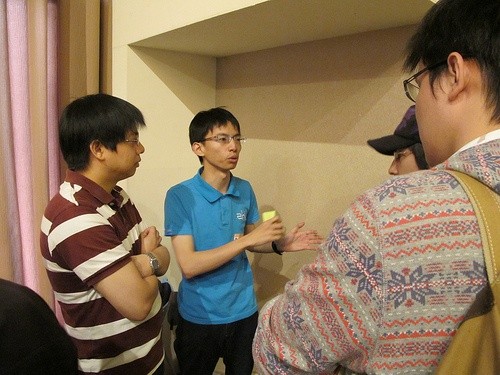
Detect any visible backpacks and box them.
[435,169,500,375]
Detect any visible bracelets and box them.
[271,241,284,255]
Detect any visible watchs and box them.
[144,252,160,276]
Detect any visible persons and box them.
[163,106,324,375]
[40,93,170,375]
[0,279,78,375]
[367,102,429,175]
[252,0,500,375]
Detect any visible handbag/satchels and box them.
[160,278,172,313]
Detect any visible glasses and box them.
[403,58,447,103]
[203,134,248,144]
[121,139,140,148]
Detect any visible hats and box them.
[367,105,419,156]
